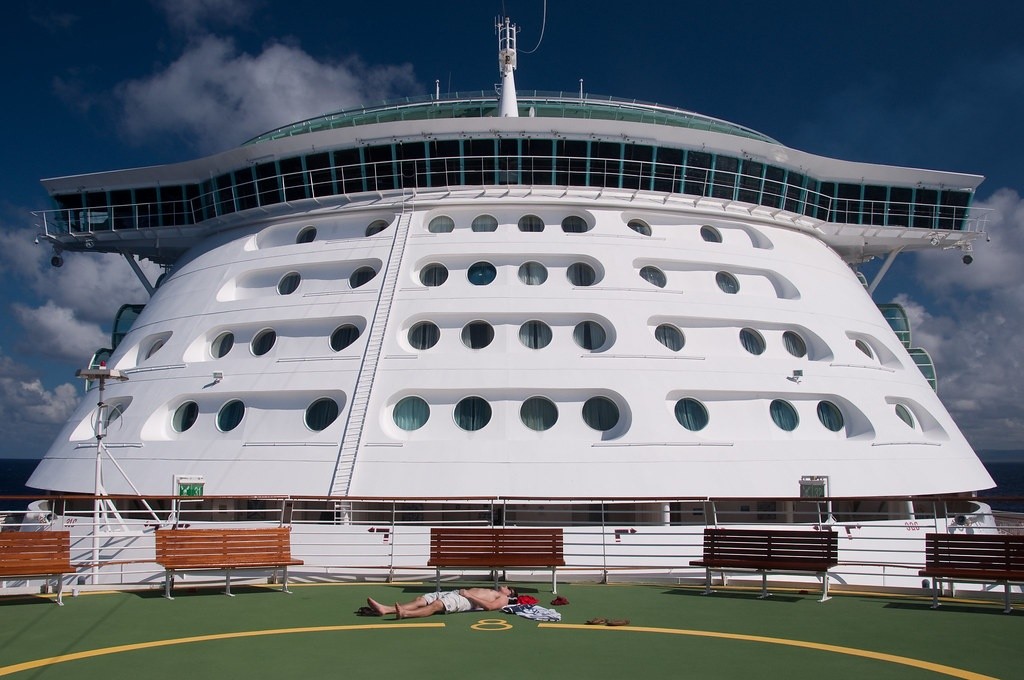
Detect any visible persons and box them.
[367,586,518,620]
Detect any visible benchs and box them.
[155,527,304,600]
[0,530,76,607]
[690,528,839,602]
[427,528,565,591]
[919,532,1024,614]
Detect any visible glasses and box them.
[508,587,512,596]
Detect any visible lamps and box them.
[961,252,974,265]
[51,251,63,267]
[85,236,95,249]
